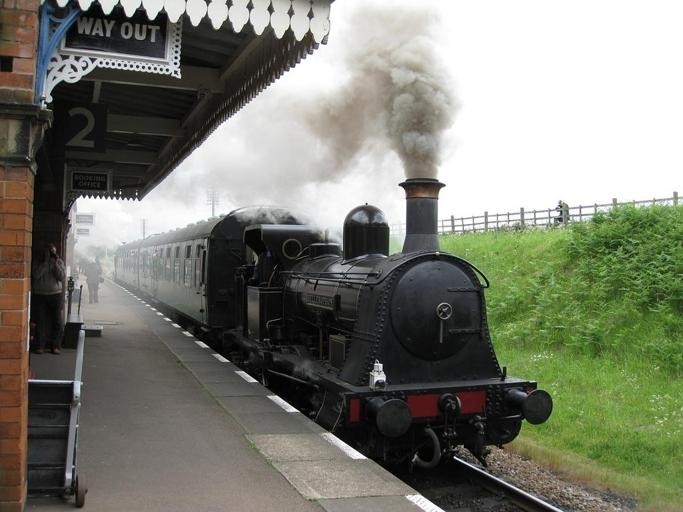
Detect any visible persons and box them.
[84,259,102,304]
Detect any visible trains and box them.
[111,175,556,475]
